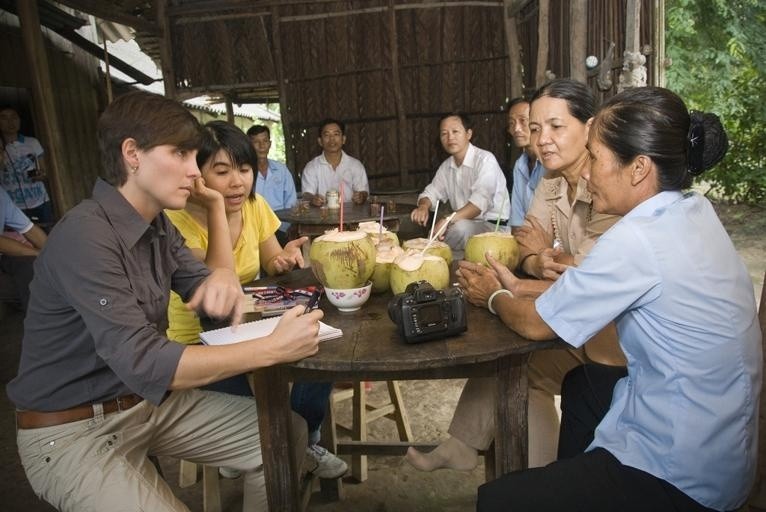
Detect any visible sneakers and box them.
[219,464,242,480]
[299,437,350,481]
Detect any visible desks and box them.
[270,199,436,257]
[367,185,420,206]
[215,259,565,511]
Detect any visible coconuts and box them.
[309,222,453,295]
[465,231,520,273]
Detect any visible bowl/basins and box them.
[323,280,372,315]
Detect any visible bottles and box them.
[326,190,338,207]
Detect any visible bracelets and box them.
[520,253,537,274]
[488,289,514,315]
[443,214,452,225]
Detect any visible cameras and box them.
[386,280,468,344]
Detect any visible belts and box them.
[16,394,146,430]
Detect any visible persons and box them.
[411,113,510,260]
[5,90,324,511]
[1,141,49,258]
[454,88,765,512]
[301,121,370,206]
[1,105,52,225]
[404,80,625,474]
[510,98,549,236]
[247,124,301,279]
[165,120,349,481]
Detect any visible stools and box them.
[328,376,414,483]
[176,455,228,512]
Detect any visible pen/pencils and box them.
[243,286,278,291]
[303,289,320,314]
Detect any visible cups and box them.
[387,199,397,214]
[372,196,381,204]
[319,206,328,220]
[371,203,381,217]
